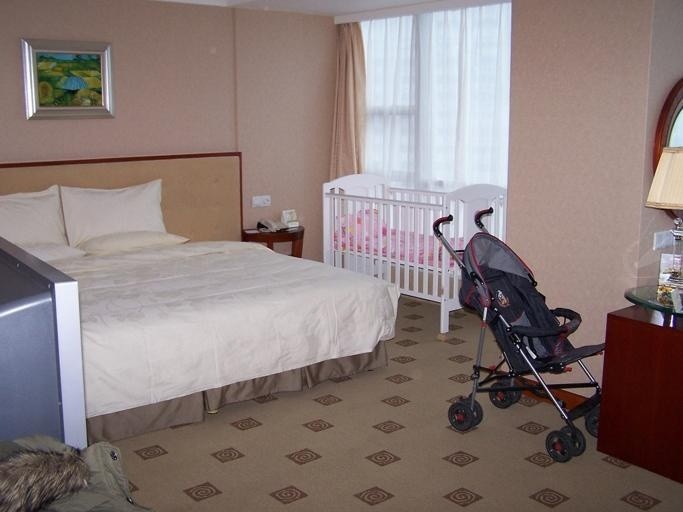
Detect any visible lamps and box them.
[644,145,683,290]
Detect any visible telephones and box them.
[257,216,288,232]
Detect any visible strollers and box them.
[433,207,609,464]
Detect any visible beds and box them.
[1,150,401,447]
[320,172,509,337]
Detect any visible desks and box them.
[242,224,306,259]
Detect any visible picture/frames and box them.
[18,37,115,121]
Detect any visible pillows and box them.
[58,175,166,248]
[333,206,390,243]
[0,183,69,250]
[15,240,87,264]
[75,229,190,256]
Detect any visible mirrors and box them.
[650,75,682,231]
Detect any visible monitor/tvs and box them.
[0,237,88,453]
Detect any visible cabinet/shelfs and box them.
[594,304,682,487]
